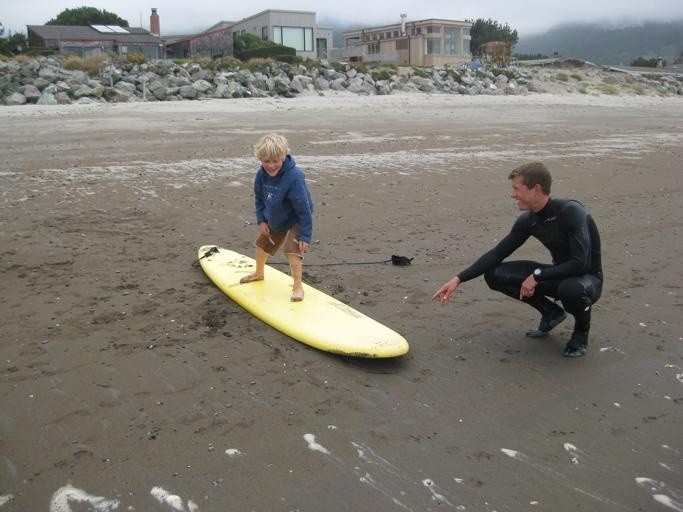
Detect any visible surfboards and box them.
[195,244,410,360]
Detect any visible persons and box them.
[429,163,605,358]
[237,131,316,301]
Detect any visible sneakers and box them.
[525,305,566,338]
[561,326,591,358]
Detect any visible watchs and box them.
[533,268,543,282]
